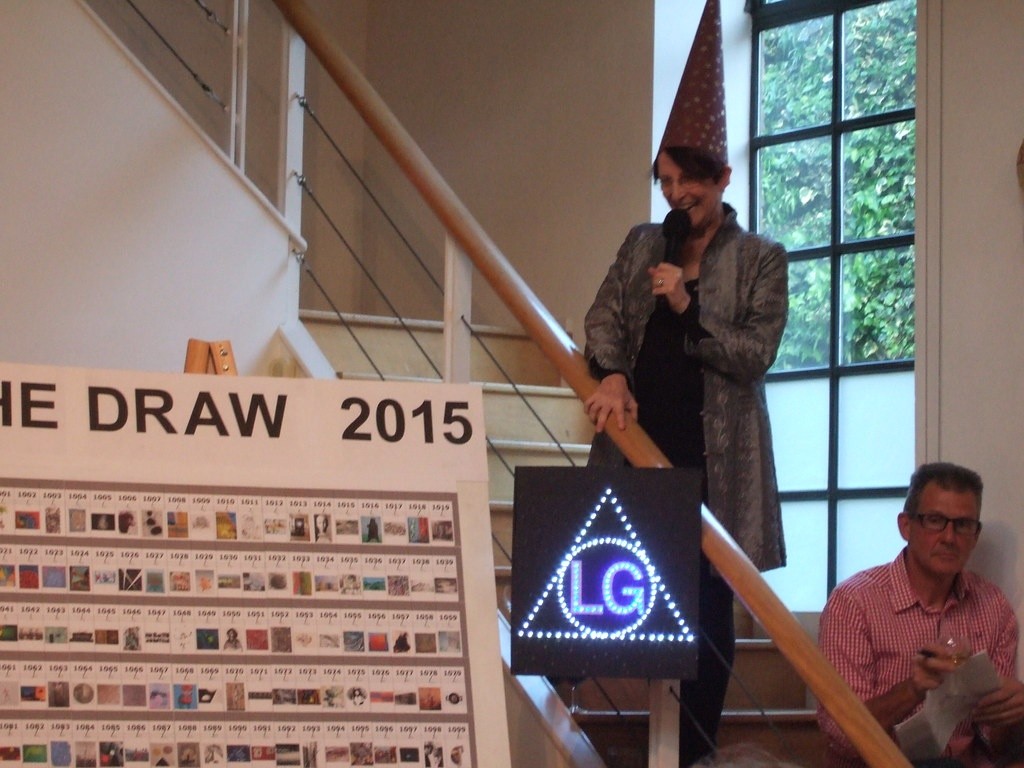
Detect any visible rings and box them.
[657,279,664,287]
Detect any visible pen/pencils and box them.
[915,647,935,660]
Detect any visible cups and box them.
[938,635,973,662]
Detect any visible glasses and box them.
[908,512,982,536]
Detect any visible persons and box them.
[817,463,1024,768]
[583,146,788,768]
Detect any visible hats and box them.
[659,0,727,166]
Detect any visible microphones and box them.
[655,208,691,309]
